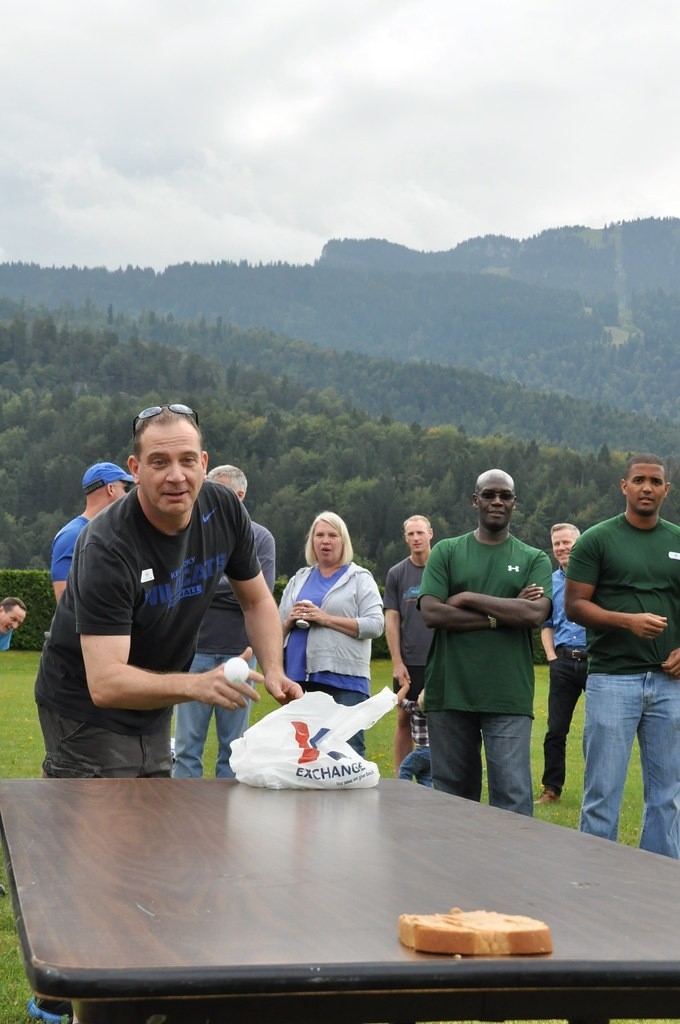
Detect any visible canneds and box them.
[296,599,313,628]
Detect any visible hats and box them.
[82,461,133,494]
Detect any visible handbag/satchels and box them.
[223,686,400,791]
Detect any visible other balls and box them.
[223,656,250,686]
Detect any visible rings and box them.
[303,613,306,615]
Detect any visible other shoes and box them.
[533,788,560,804]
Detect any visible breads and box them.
[398,907,553,955]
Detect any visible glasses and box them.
[481,491,514,501]
[132,403,199,429]
[125,486,131,494]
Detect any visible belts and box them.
[557,646,587,660]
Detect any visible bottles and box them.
[296,599,313,629]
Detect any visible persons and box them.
[173,464,276,780]
[383,515,434,777]
[536,523,588,803]
[0,597,27,651]
[51,463,136,605]
[28,404,304,1024]
[417,469,553,816]
[397,677,432,788]
[278,511,385,757]
[564,456,680,859]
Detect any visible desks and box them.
[0,776,680,1024]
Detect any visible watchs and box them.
[488,615,496,628]
